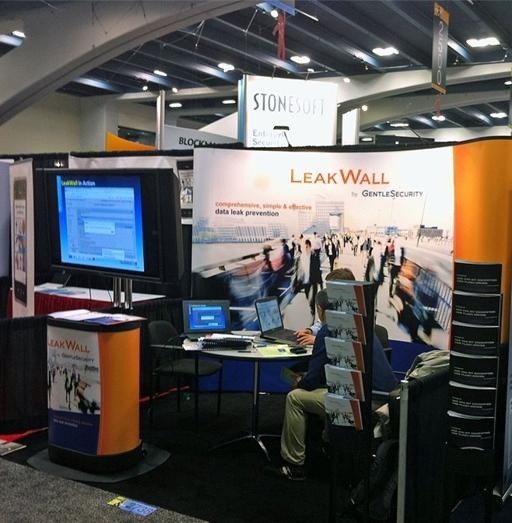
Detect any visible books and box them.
[82,311,146,325]
[322,279,368,430]
[41,308,109,320]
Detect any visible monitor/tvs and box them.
[35,167,186,287]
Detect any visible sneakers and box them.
[272,463,304,480]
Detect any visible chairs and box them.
[141,320,222,437]
[364,322,449,455]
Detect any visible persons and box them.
[278,229,434,347]
[46,361,80,411]
[265,289,399,481]
[293,266,358,347]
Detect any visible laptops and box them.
[254,296,303,347]
[182,299,233,342]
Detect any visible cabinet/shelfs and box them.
[314,278,377,522]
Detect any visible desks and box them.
[180,326,316,469]
